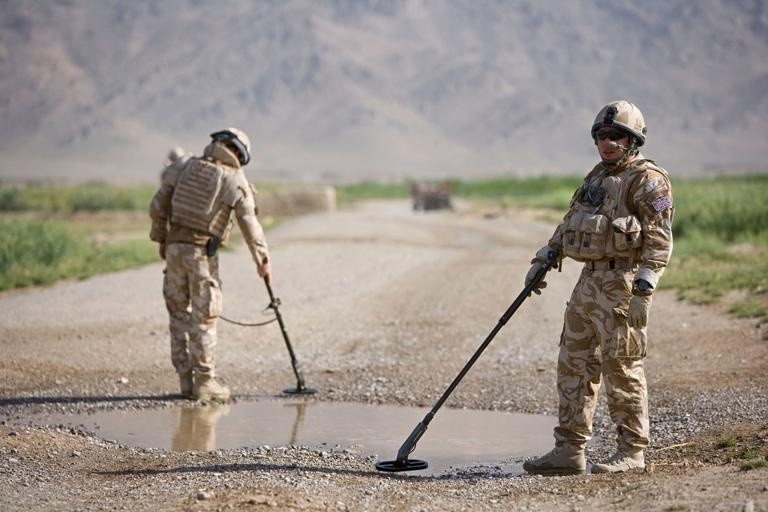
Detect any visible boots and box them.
[177,367,232,401]
[591,436,646,475]
[523,438,586,475]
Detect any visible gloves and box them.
[626,287,653,331]
[523,256,548,298]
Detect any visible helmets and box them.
[591,99,647,146]
[209,126,252,166]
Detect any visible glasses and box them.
[595,129,627,142]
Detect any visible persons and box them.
[147,125,272,401]
[518,97,675,478]
